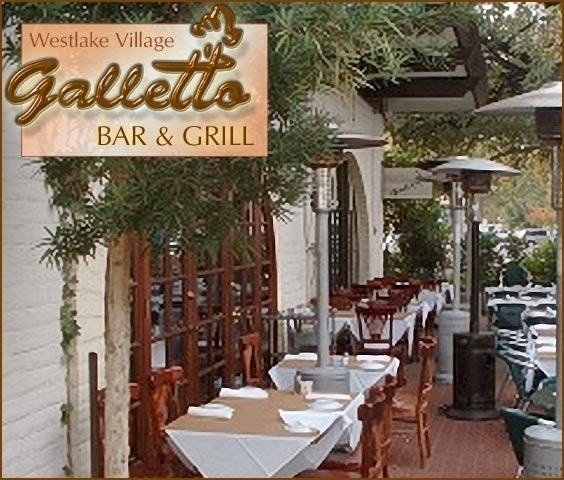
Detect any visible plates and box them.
[359,362,386,372]
[310,398,344,412]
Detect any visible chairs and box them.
[483,259,564,480]
[88,266,455,479]
